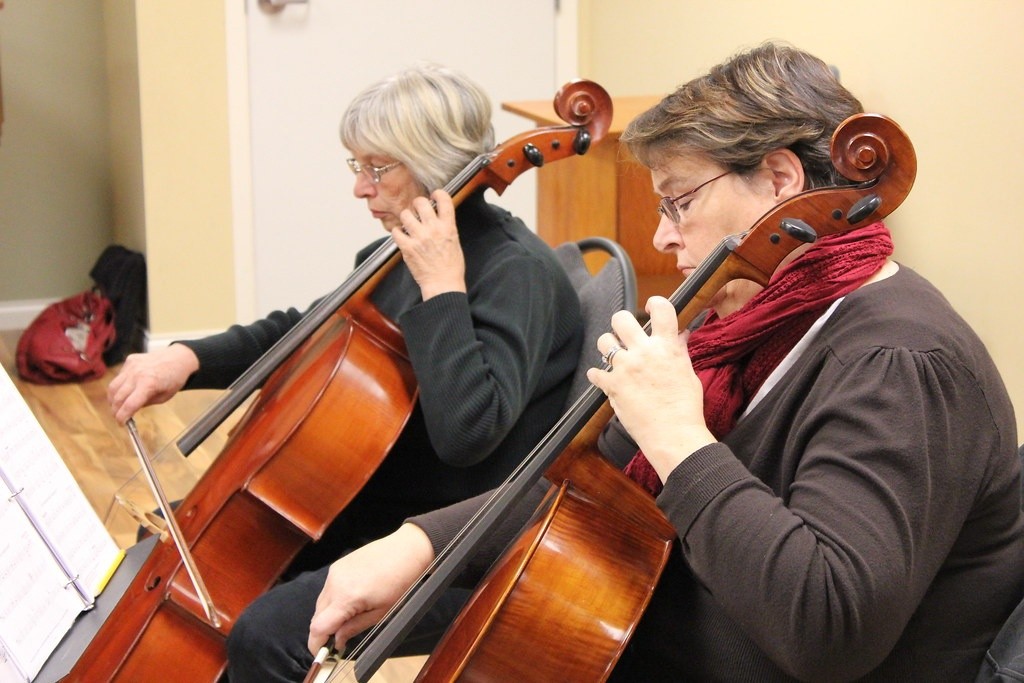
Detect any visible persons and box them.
[107,64,586,683]
[306,38,1023,683]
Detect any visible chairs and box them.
[334,236,639,662]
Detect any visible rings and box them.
[601,344,625,364]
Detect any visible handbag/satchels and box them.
[89,244,146,367]
[17,287,117,384]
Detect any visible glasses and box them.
[346,157,403,183]
[655,163,753,223]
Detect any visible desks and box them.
[502,95,688,318]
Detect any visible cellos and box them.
[42,77,617,681]
[299,113,918,681]
